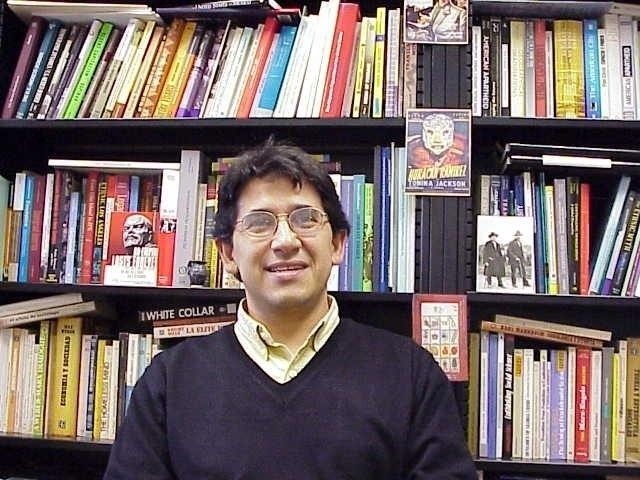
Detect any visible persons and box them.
[101,137,482,480]
[417,0,467,42]
[412,113,465,171]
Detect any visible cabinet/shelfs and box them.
[0,0,640,478]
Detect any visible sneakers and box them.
[488,281,531,288]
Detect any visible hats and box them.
[512,230,523,236]
[489,232,498,238]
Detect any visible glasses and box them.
[231,207,329,239]
